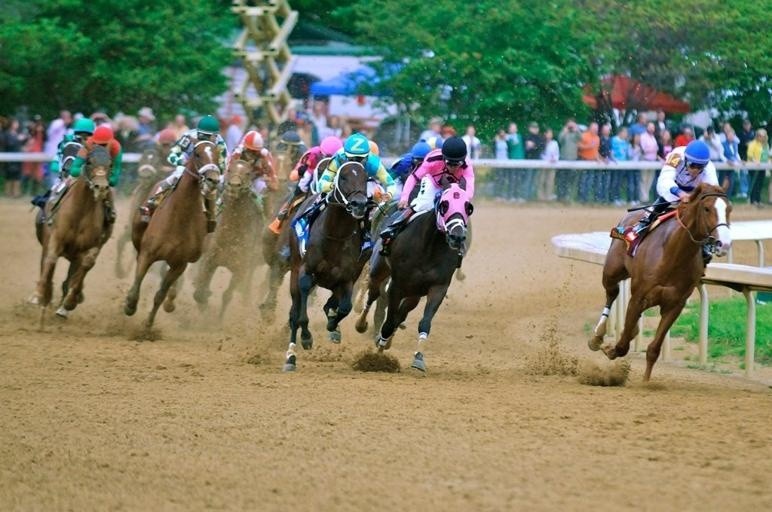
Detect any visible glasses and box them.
[687,162,705,170]
[346,156,364,162]
[444,159,465,167]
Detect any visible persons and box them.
[627,142,720,261]
[490,117,772,209]
[2,100,481,261]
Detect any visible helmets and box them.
[158,129,177,144]
[684,140,711,165]
[279,131,303,146]
[242,130,264,151]
[320,136,343,157]
[411,142,432,159]
[91,126,113,144]
[368,140,379,156]
[442,136,467,160]
[343,133,370,158]
[196,115,221,134]
[70,118,96,135]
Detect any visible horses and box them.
[116,143,169,279]
[27,139,117,320]
[259,191,315,325]
[192,157,290,321]
[239,147,299,308]
[124,134,221,332]
[284,154,374,372]
[352,261,393,341]
[588,177,732,383]
[355,175,474,372]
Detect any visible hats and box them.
[136,106,155,120]
